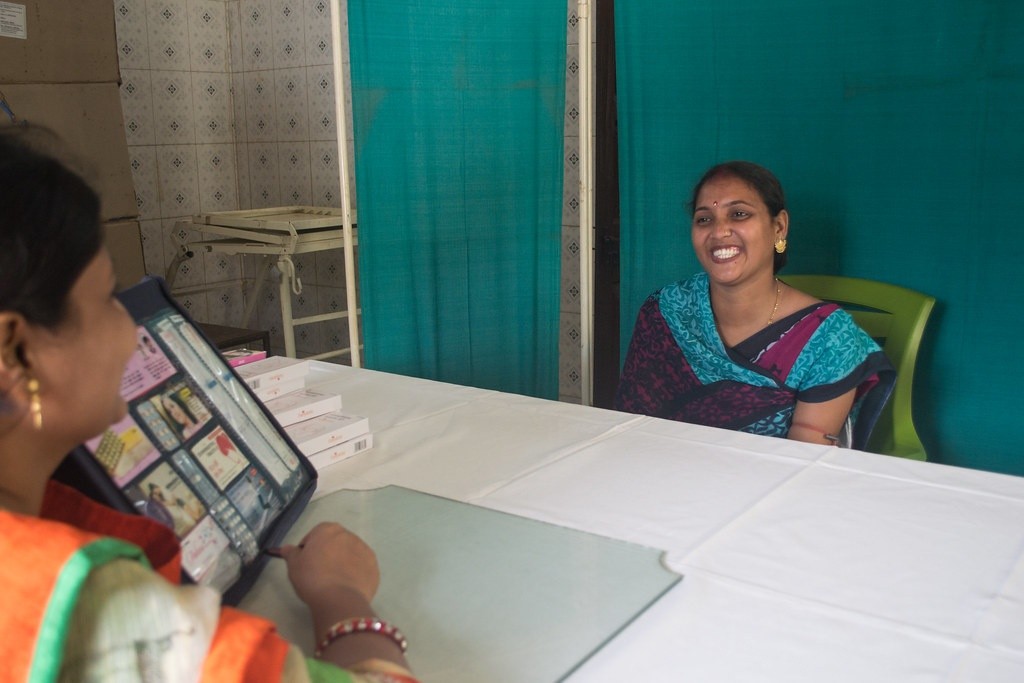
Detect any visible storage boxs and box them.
[222,348,373,470]
[0,0,147,294]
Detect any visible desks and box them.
[235,355,1024,683]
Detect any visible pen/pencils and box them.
[264,545,304,559]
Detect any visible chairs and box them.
[776,275,936,462]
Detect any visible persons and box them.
[623,161,888,449]
[0,112,424,683]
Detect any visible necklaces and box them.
[767,275,782,326]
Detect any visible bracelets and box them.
[312,616,408,660]
